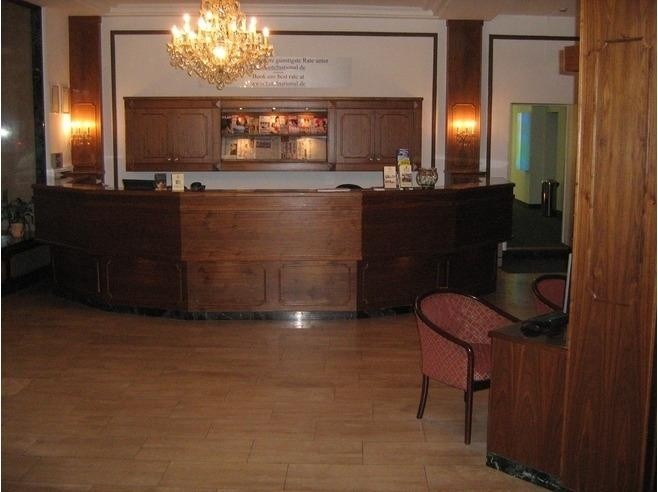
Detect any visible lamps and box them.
[165,1,275,92]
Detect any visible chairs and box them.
[414,274,569,446]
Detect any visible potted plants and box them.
[1,197,34,239]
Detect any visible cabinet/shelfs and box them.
[335,110,412,163]
[133,109,212,163]
[222,109,327,163]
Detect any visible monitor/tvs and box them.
[120,179,158,190]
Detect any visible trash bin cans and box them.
[541,179,559,217]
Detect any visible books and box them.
[217,112,328,161]
[381,147,415,190]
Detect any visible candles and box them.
[70,127,91,135]
[456,126,475,135]
